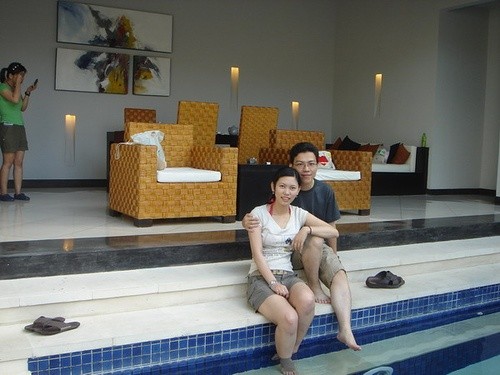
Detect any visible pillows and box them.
[330,135,411,164]
[130,130,166,170]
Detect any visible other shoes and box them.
[14,193,30,201]
[0,194,14,201]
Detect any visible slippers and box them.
[34,321,80,335]
[24,316,66,332]
[366,271,405,288]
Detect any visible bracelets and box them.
[24,91,30,96]
[306,224,312,235]
[269,281,279,287]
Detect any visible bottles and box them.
[421,132,426,147]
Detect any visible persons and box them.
[242,142,361,351]
[0,62,38,202]
[246,166,340,375]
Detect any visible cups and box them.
[228,125,238,136]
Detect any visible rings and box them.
[282,289,284,291]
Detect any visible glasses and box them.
[292,161,317,168]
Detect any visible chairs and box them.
[106,100,373,226]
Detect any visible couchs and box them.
[326,142,429,196]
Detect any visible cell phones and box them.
[34,79,39,86]
